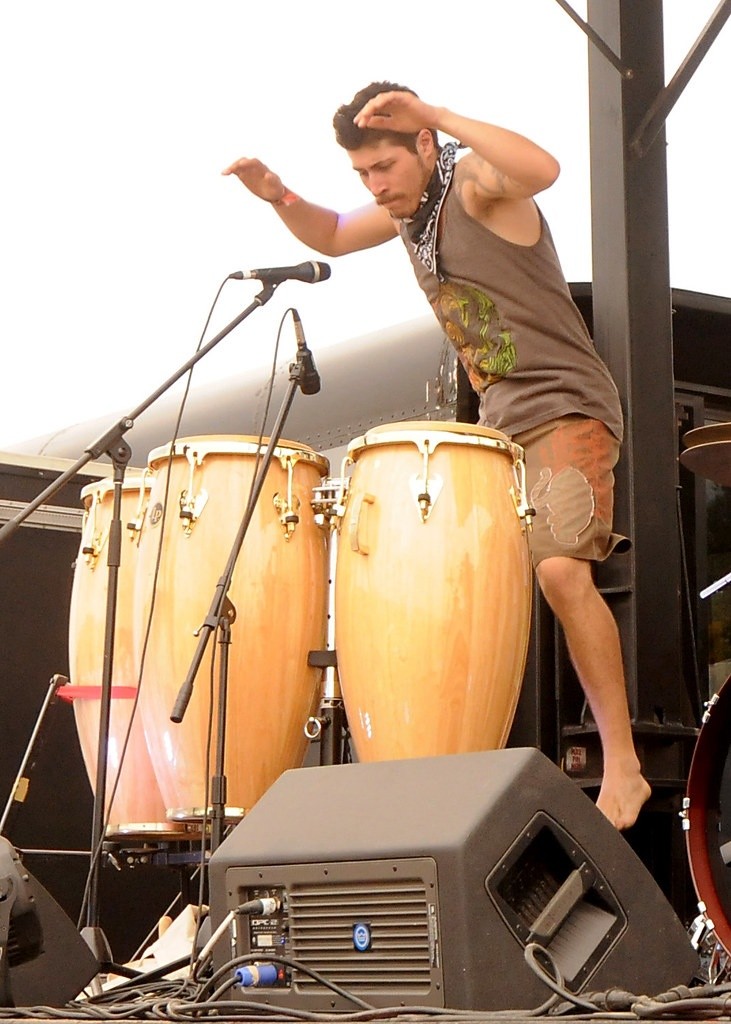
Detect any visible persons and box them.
[220,82,653,833]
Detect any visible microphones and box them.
[229,261,331,284]
[292,309,321,395]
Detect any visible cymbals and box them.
[679,440,731,486]
[682,422,731,448]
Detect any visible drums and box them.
[64,470,209,843]
[326,415,536,764]
[134,436,331,823]
[677,668,731,987]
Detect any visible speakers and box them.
[0,834,101,1012]
[208,745,701,1020]
[558,722,700,927]
